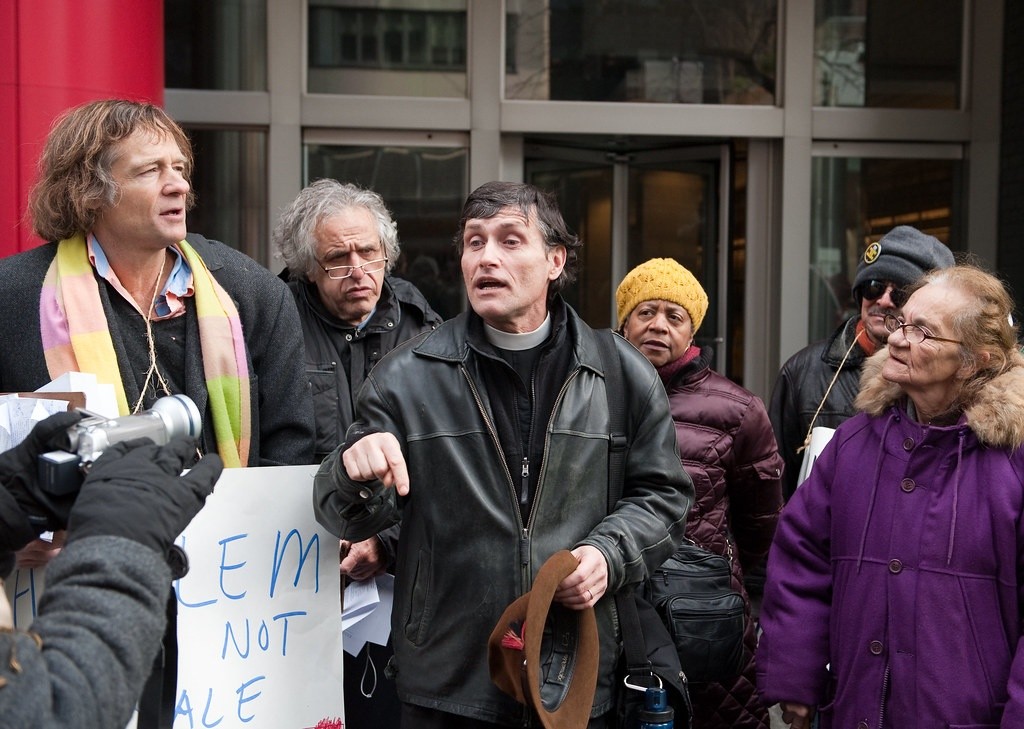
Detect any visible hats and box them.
[487,549,598,728]
[853,225,955,295]
[616,256,709,336]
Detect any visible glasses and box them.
[859,278,909,309]
[314,239,389,280]
[884,313,967,346]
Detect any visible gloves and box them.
[63,439,223,580]
[0,413,85,554]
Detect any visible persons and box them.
[757,266,1023,728]
[1,98,320,729]
[768,223,958,511]
[615,257,788,729]
[0,411,225,728]
[310,181,698,728]
[276,176,448,729]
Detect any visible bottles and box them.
[623,671,674,729]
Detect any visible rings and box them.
[587,589,595,602]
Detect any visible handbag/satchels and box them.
[620,595,696,728]
[644,546,747,684]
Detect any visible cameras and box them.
[37,393,200,497]
